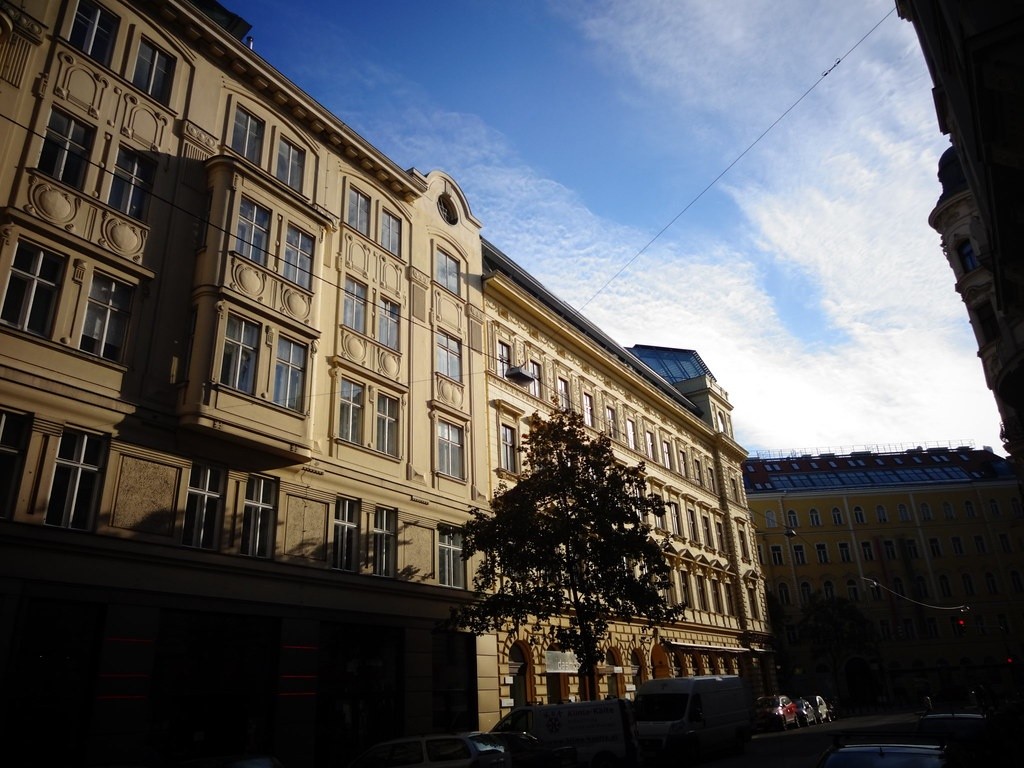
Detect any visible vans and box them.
[491,698,643,768]
[632,674,753,765]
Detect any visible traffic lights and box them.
[1005,656,1015,671]
[957,619,967,634]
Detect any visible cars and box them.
[791,698,817,726]
[912,702,996,768]
[802,695,832,724]
[347,730,513,768]
[165,754,286,768]
[749,694,801,732]
[490,731,578,768]
[814,732,986,768]
[824,697,842,720]
